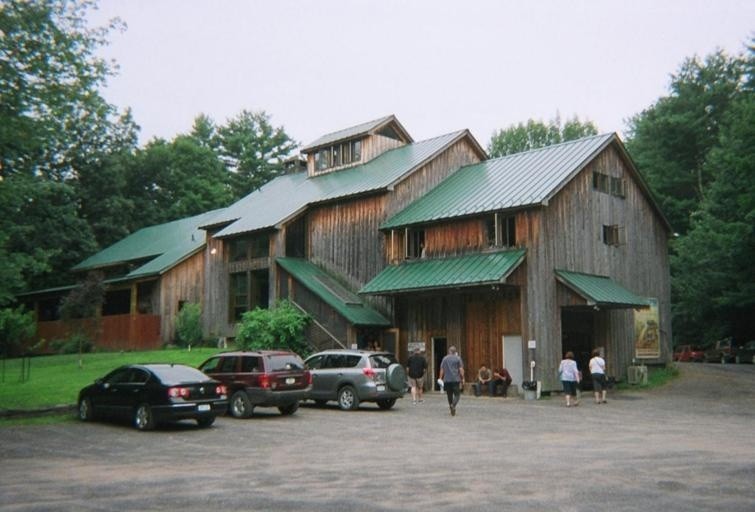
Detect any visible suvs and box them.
[300,350,409,410]
[198,347,313,417]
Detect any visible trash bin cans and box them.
[522,380,537,401]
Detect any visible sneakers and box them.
[450,403,456,416]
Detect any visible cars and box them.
[673,338,755,364]
[77,362,229,430]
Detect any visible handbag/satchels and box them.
[605,374,609,382]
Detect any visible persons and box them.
[588,347,609,405]
[438,345,464,416]
[557,349,582,407]
[492,365,513,398]
[419,240,427,260]
[473,365,494,397]
[405,346,428,405]
[365,340,382,352]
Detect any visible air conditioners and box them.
[628,365,648,384]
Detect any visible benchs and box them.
[462,381,518,398]
